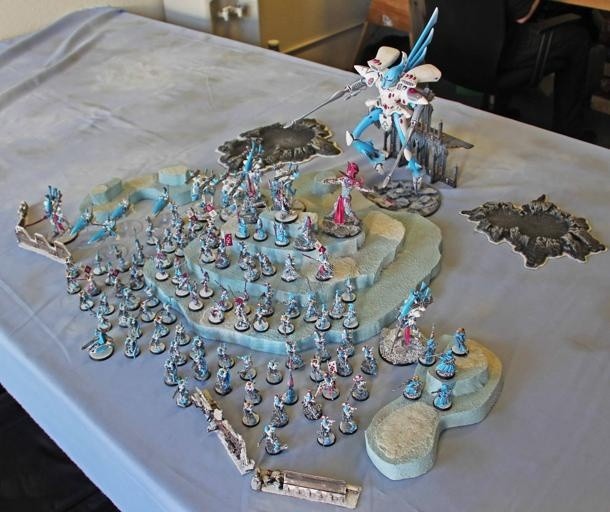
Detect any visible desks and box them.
[0,7,610,512]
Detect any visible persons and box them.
[345,46,441,191]
[16,137,468,456]
